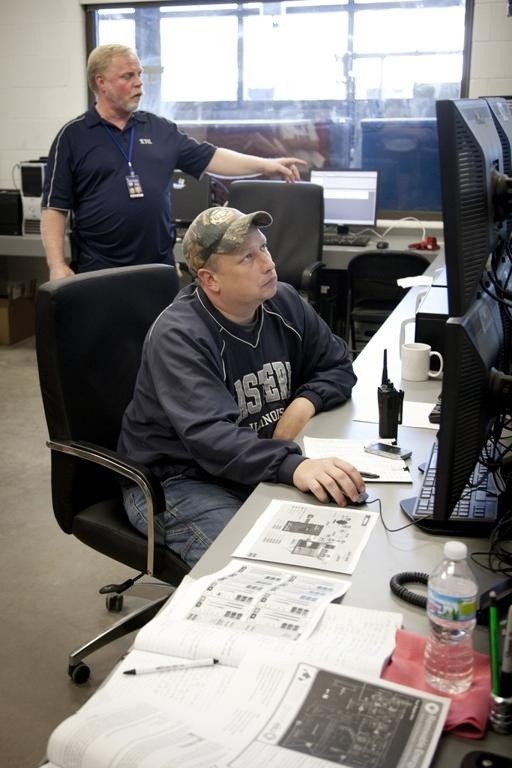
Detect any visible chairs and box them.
[227,180,340,338]
[343,251,429,358]
[35,263,195,686]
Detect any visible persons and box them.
[114,202,369,577]
[35,40,312,282]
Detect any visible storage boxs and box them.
[0,256,71,346]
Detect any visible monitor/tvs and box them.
[307,168,379,233]
[433,95,512,526]
[171,166,211,224]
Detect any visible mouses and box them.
[377,241,389,249]
[329,488,369,506]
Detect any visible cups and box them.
[400,343,444,383]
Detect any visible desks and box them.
[0,229,512,768]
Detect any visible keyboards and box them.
[323,233,371,247]
[412,438,503,527]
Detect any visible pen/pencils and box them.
[489,605,512,698]
[360,472,380,479]
[123,659,219,675]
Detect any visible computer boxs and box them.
[19,160,49,236]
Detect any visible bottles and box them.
[421,541,480,694]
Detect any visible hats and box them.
[182,204,276,280]
